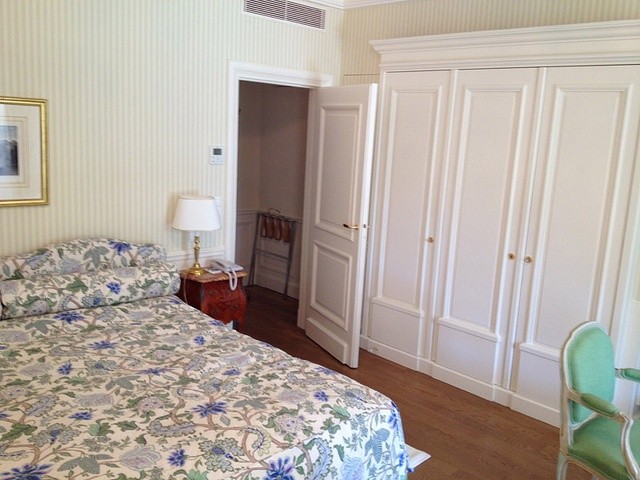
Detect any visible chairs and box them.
[556,319,640,477]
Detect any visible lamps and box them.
[173,195,222,277]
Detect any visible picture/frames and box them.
[1,97,52,209]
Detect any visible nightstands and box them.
[181,268,248,333]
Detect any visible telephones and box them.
[208,258,244,291]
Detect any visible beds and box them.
[0,238,408,480]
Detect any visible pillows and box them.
[2,263,185,320]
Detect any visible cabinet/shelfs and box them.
[358,27,452,370]
[599,260,606,263]
[402,21,640,420]
